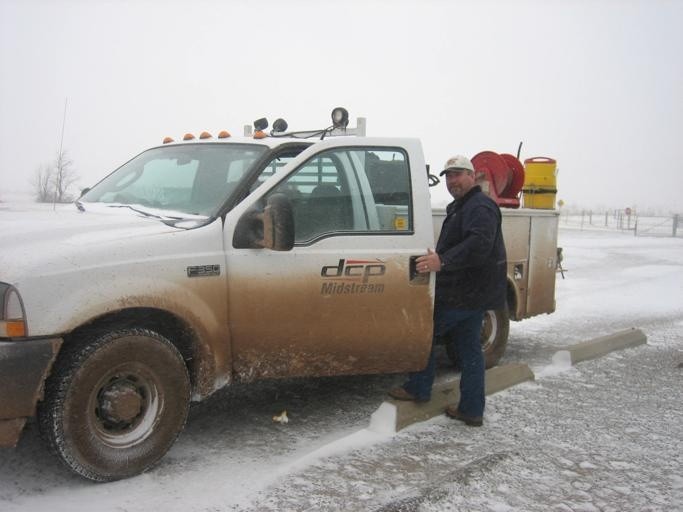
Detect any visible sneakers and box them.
[447,405,483,426]
[389,388,430,401]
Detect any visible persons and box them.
[387,155,512,427]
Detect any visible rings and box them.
[423,263,430,272]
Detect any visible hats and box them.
[440,155,474,176]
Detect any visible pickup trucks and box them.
[0,110,561,482]
